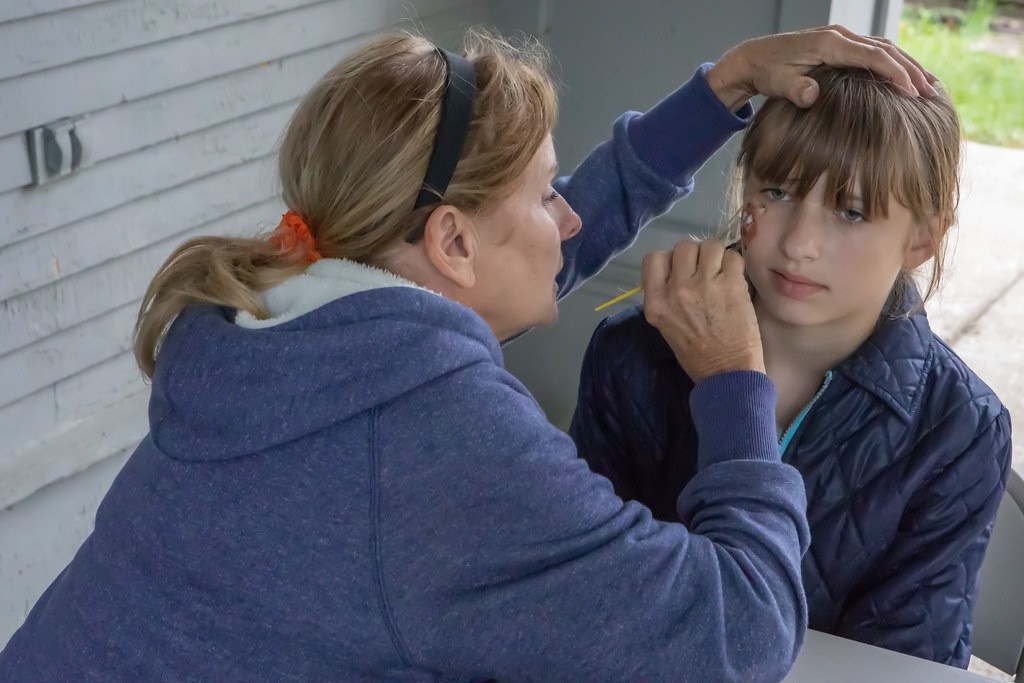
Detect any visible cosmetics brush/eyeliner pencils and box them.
[594,239,746,313]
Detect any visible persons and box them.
[567,65,1012,670]
[0,23,945,683]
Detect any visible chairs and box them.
[972,467,1024,683]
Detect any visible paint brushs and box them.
[594,236,746,312]
